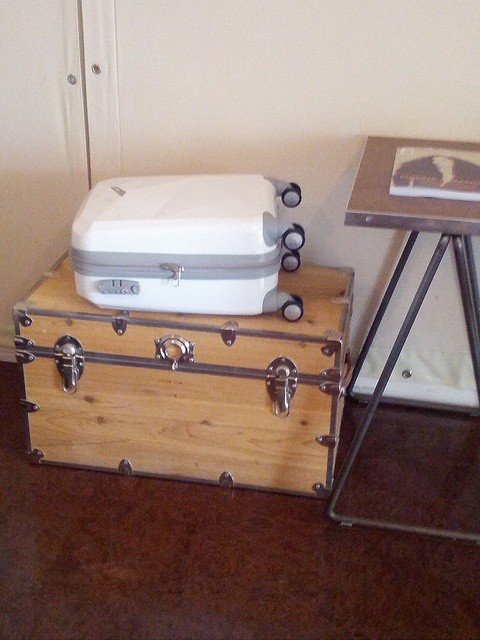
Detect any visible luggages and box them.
[71,175,304,324]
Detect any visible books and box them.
[390,146,480,202]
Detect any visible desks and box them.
[326,135,480,544]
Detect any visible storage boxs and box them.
[11,251,356,502]
